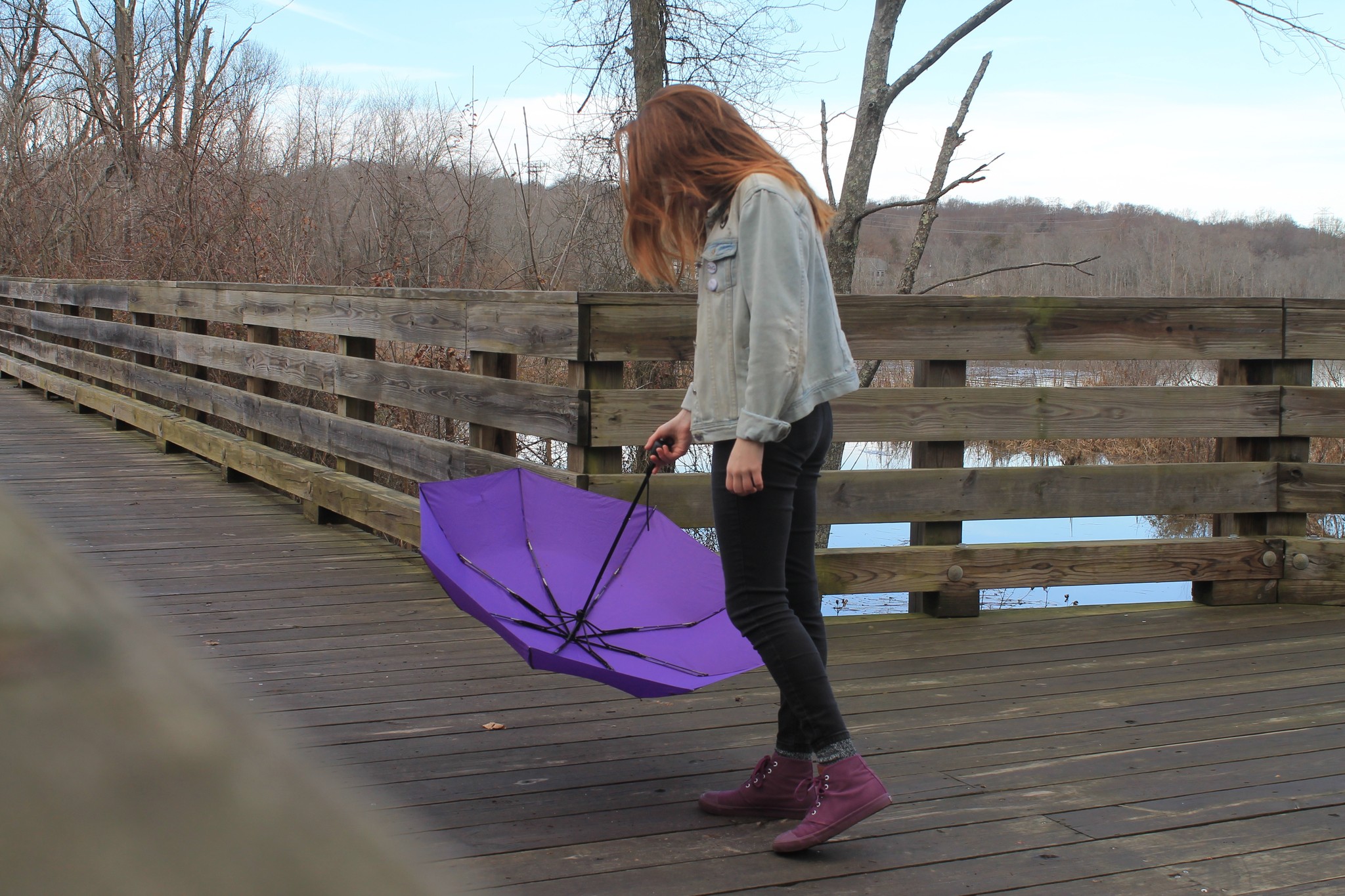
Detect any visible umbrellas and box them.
[418,437,765,699]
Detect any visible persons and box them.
[614,84,892,853]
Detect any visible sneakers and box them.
[699,752,816,820]
[772,753,893,852]
